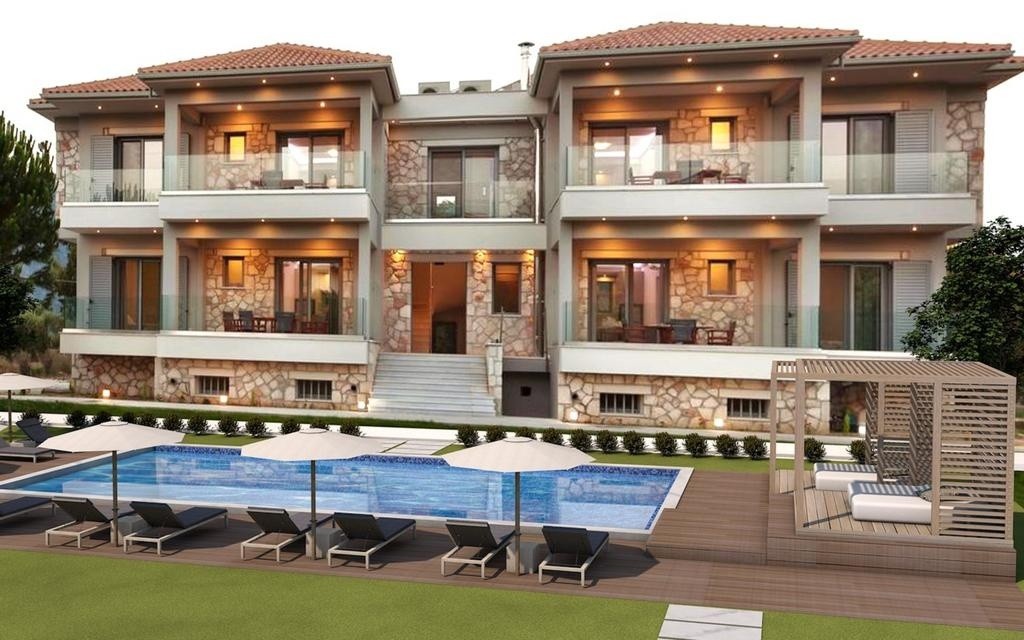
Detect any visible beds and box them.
[813,462,978,523]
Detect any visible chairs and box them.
[275,312,293,334]
[239,311,254,332]
[628,166,654,186]
[16,418,53,443]
[0,435,56,464]
[222,312,235,331]
[262,171,283,188]
[599,319,736,346]
[228,179,248,189]
[305,174,327,189]
[0,495,610,589]
[722,163,749,183]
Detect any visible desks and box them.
[251,178,304,189]
[236,316,288,333]
[655,169,721,184]
[641,324,713,344]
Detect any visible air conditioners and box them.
[418,82,450,94]
[459,80,491,93]
